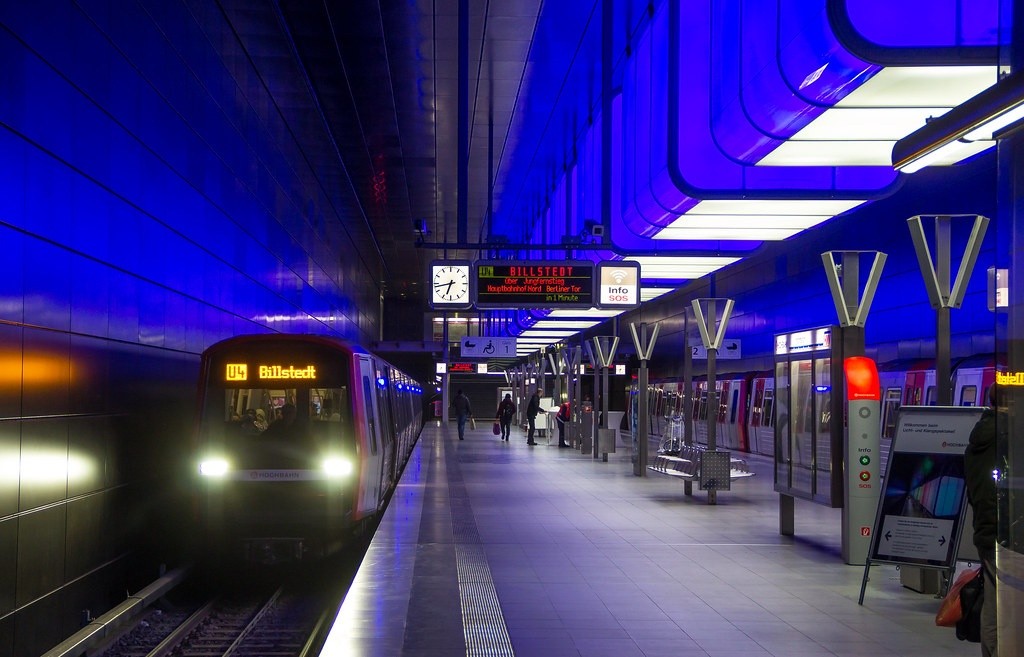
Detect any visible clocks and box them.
[433,264,470,305]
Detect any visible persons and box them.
[230,400,319,445]
[495,392,517,440]
[452,388,473,440]
[556,399,576,448]
[963,375,1008,657]
[526,388,548,445]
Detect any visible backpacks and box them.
[502,403,512,419]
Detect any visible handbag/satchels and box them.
[493,418,500,435]
[470,417,476,430]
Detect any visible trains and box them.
[183,330,428,579]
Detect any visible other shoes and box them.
[505,435,508,441]
[559,445,569,448]
[459,436,464,440]
[501,434,505,439]
[527,441,537,445]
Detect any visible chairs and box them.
[647,437,755,481]
